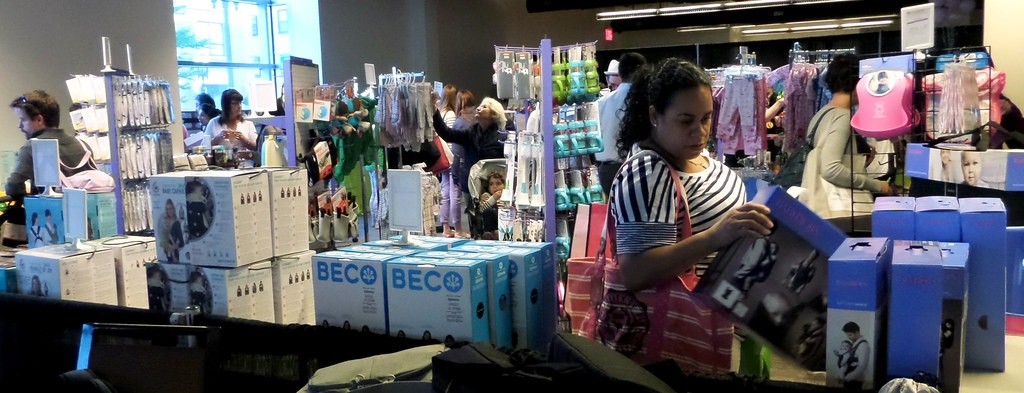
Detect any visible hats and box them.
[604,59,619,76]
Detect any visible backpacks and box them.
[29,136,116,194]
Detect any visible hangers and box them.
[789,46,855,67]
[725,53,770,81]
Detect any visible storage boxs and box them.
[7,140,1024,393]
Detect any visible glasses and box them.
[20,95,33,108]
[230,100,244,107]
[608,80,619,87]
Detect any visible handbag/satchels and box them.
[567,150,734,377]
[798,108,874,218]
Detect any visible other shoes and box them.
[455,232,471,239]
[483,232,495,240]
[443,230,455,237]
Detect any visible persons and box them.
[435,85,471,238]
[595,53,647,199]
[600,59,622,96]
[479,172,505,240]
[27,275,48,297]
[964,44,1024,149]
[796,316,827,365]
[159,198,185,263]
[934,148,990,188]
[182,89,258,160]
[765,96,784,123]
[833,321,870,388]
[730,227,819,302]
[5,90,96,203]
[29,209,59,247]
[452,89,478,238]
[806,52,893,237]
[433,97,508,236]
[609,57,774,382]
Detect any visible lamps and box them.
[596,0,894,38]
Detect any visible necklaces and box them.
[687,158,707,169]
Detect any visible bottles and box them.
[186,304,200,326]
[261,135,284,167]
[192,145,228,165]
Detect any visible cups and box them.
[552,60,606,261]
